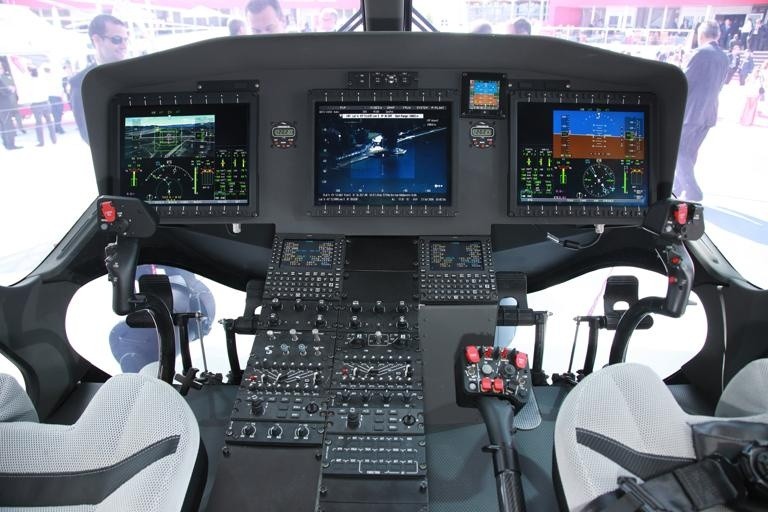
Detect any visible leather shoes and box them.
[4,125,66,150]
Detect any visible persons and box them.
[670,20,729,202]
[246,0,285,35]
[227,18,254,36]
[69,13,130,146]
[319,8,339,32]
[555,16,768,126]
[1,61,72,150]
[507,16,531,35]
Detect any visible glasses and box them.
[100,35,128,45]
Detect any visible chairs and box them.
[0,371,207,512]
[552,359,768,512]
